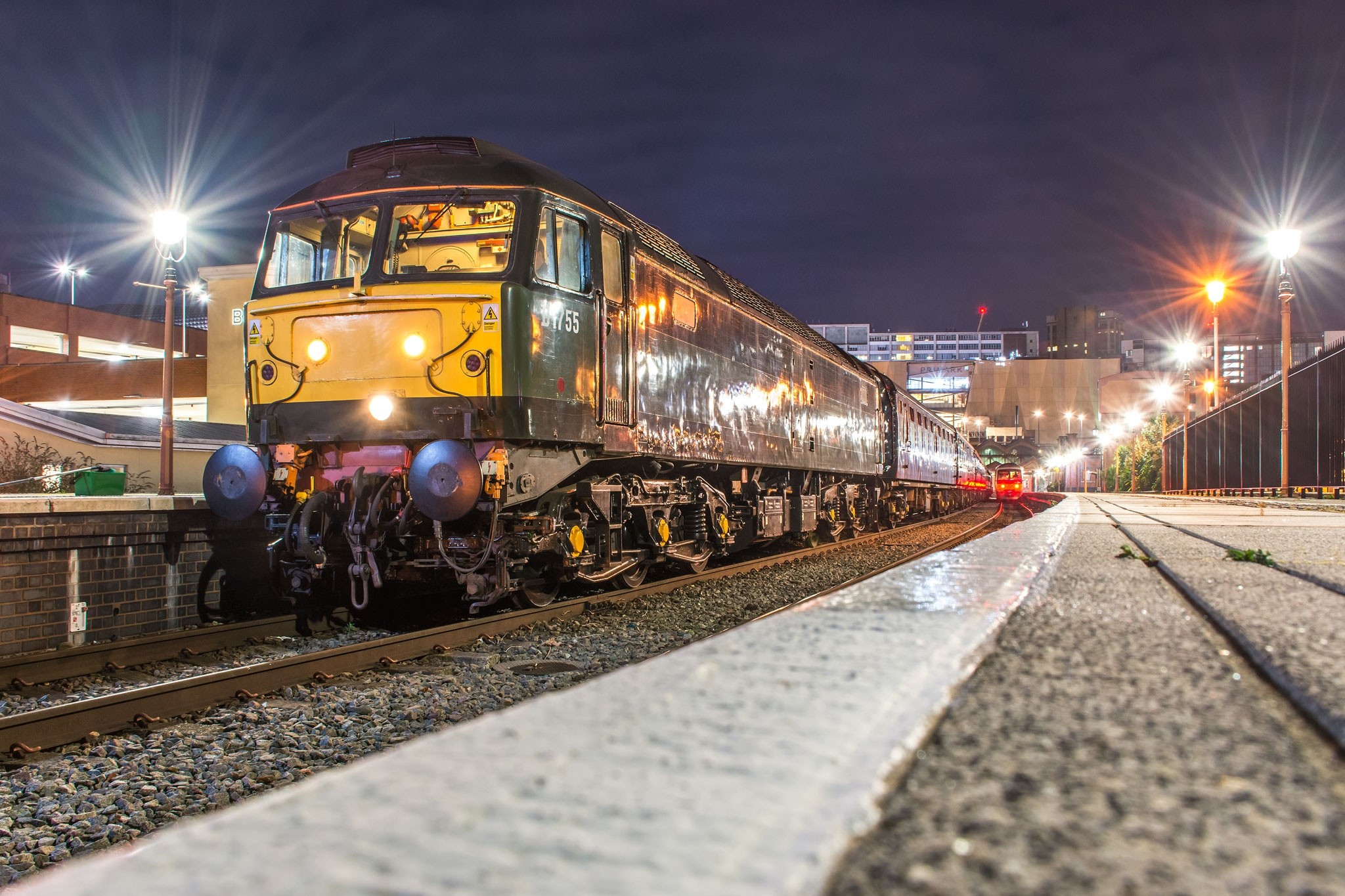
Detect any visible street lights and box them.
[1032,227,1302,496]
[150,208,189,496]
[59,266,87,305]
[175,284,199,357]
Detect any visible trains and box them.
[995,463,1025,502]
[202,135,994,616]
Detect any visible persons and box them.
[1012,471,1019,478]
[534,237,548,279]
